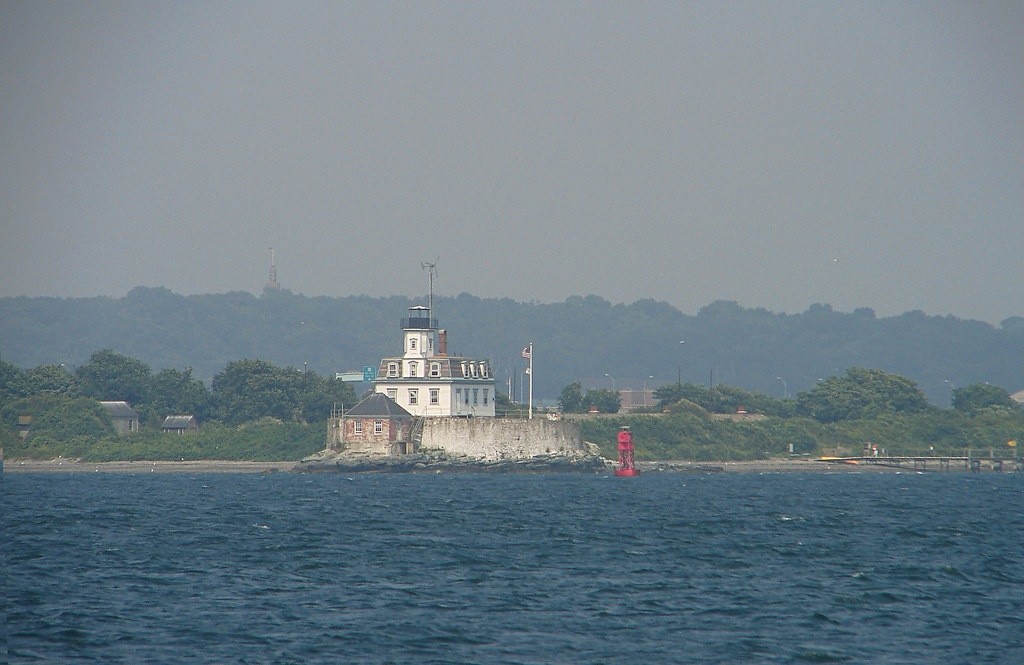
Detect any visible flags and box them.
[1007,438,1016,447]
[525,368,530,375]
[521,346,530,359]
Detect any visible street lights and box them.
[643,376,654,409]
[943,378,956,410]
[604,373,615,393]
[777,376,787,398]
